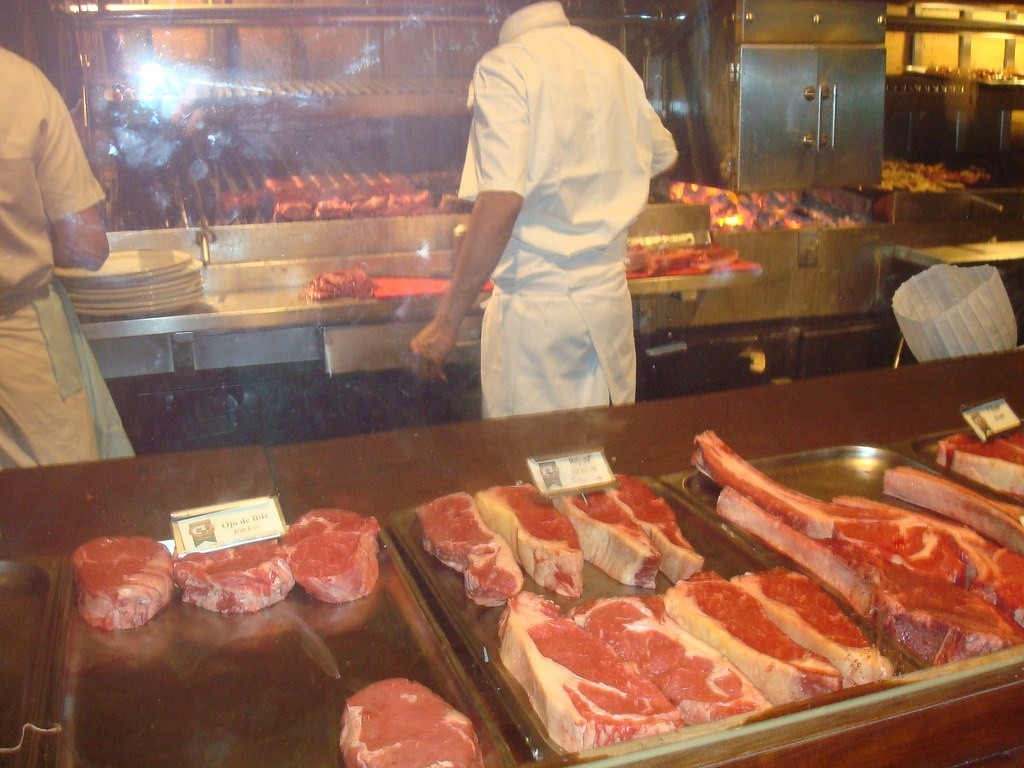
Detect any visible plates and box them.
[53,250,205,316]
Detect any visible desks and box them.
[0,350,1024,768]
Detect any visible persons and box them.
[408,0,680,419]
[0,46,135,468]
